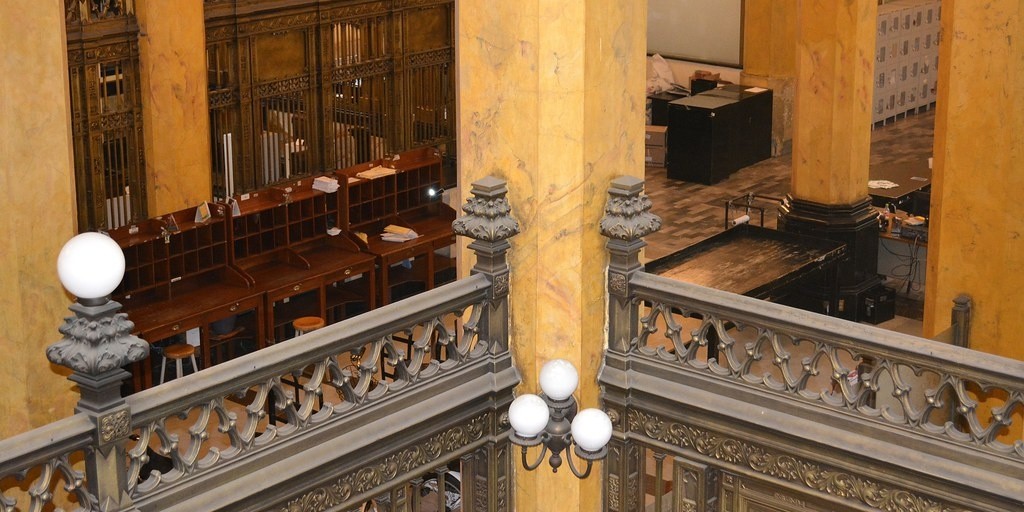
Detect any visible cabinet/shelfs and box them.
[97,147,443,301]
[871,0,941,131]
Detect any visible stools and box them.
[292,317,325,337]
[160,344,199,385]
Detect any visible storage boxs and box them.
[645,125,668,168]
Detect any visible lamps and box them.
[45,231,151,413]
[508,359,613,480]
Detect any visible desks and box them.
[644,223,848,366]
[237,237,380,347]
[868,161,932,214]
[646,87,691,126]
[119,325,142,394]
[667,84,773,186]
[115,270,267,390]
[335,212,456,306]
